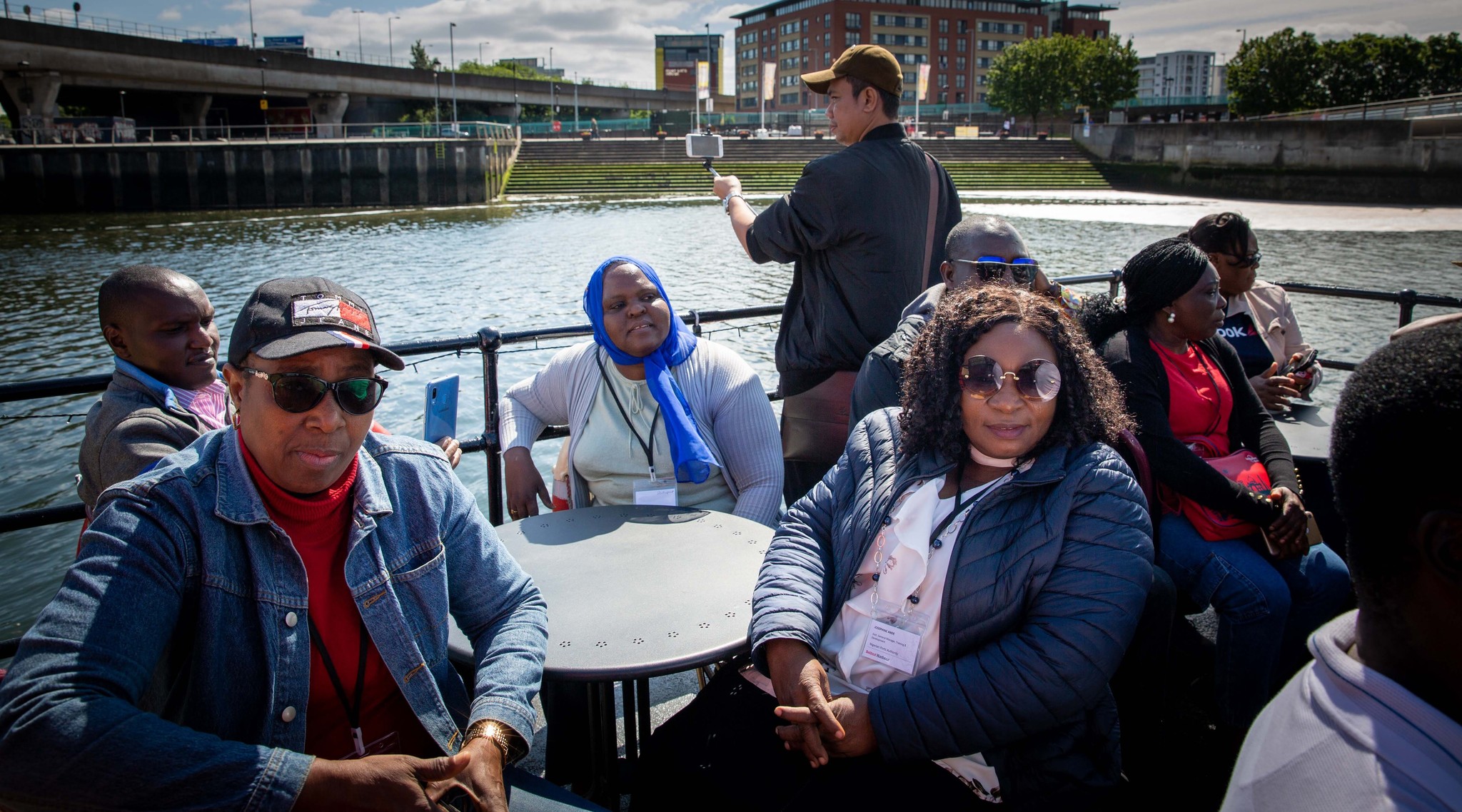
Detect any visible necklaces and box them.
[967,442,1027,467]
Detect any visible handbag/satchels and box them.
[1157,435,1272,542]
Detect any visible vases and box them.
[999,134,1009,139]
[814,134,824,140]
[740,134,749,140]
[1038,135,1048,139]
[936,134,946,138]
[656,134,666,140]
[580,135,590,141]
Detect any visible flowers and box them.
[812,130,825,135]
[580,132,591,135]
[935,130,946,134]
[998,131,1009,135]
[656,131,667,135]
[1036,131,1048,135]
[740,131,749,135]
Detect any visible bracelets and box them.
[1042,279,1059,301]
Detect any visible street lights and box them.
[705,23,710,126]
[353,10,364,64]
[388,16,401,67]
[965,28,974,125]
[120,91,126,118]
[1236,29,1246,44]
[450,22,457,138]
[550,47,554,122]
[479,41,489,65]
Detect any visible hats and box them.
[227,276,405,371]
[800,44,904,95]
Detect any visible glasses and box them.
[1219,253,1262,269]
[960,354,1064,404]
[948,256,1041,284]
[237,364,390,413]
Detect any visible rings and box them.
[510,509,518,514]
[1299,384,1303,391]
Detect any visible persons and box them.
[1220,315,1462,812]
[499,258,785,535]
[636,284,1153,812]
[77,264,464,526]
[1112,237,1356,798]
[846,214,1130,440]
[1176,212,1321,411]
[0,276,616,812]
[712,43,962,511]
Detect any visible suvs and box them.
[435,128,469,138]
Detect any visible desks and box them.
[448,505,776,812]
[1270,391,1338,463]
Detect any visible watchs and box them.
[723,191,745,216]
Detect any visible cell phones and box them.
[1258,511,1324,555]
[1280,349,1319,377]
[435,782,486,812]
[685,133,723,158]
[423,373,460,445]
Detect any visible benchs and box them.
[768,131,788,137]
[978,131,994,137]
[909,132,927,137]
